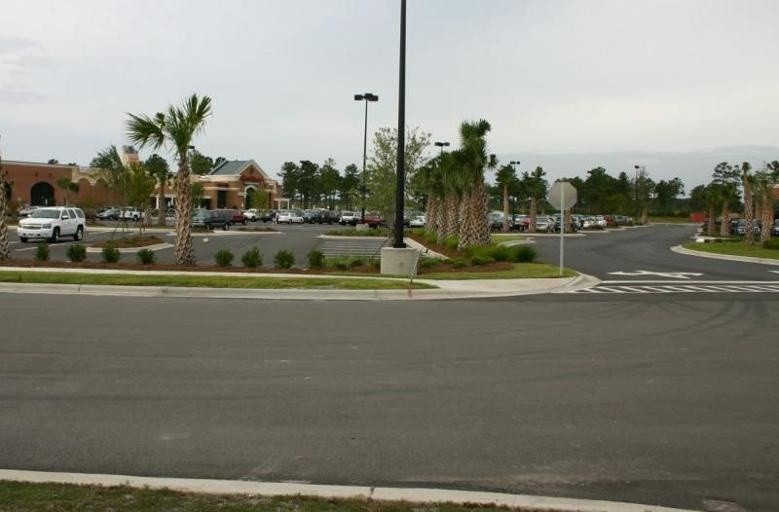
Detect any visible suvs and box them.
[16,205,89,242]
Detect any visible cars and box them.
[488,212,633,234]
[95,205,148,221]
[191,201,423,231]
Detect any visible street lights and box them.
[353,92,379,230]
[510,160,520,221]
[436,140,450,158]
